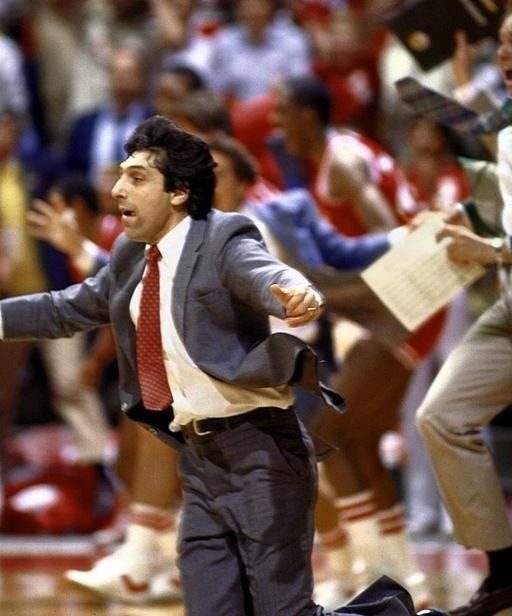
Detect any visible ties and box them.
[137,245,173,411]
[393,75,507,134]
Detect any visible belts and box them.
[185,413,252,439]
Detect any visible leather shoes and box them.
[448,577,511,614]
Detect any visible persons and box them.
[1,1,511,615]
